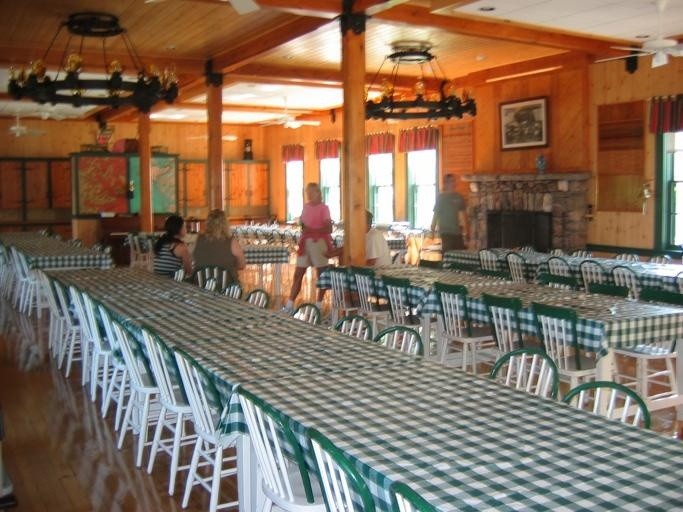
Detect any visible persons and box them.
[282,182,334,316]
[193,208,246,291]
[324,211,393,310]
[151,215,192,280]
[428,173,470,260]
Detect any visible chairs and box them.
[611,265,643,299]
[177,353,237,512]
[136,238,146,267]
[71,238,83,247]
[433,283,494,376]
[193,267,234,295]
[520,246,533,252]
[10,246,27,309]
[447,263,476,274]
[382,275,437,352]
[479,270,505,281]
[19,254,45,317]
[614,288,683,420]
[674,271,682,294]
[418,260,444,267]
[594,284,628,298]
[81,292,113,403]
[561,381,650,429]
[353,265,390,338]
[292,303,321,325]
[202,277,216,291]
[240,388,348,511]
[579,260,610,293]
[548,256,574,276]
[38,270,64,356]
[143,331,219,496]
[507,252,529,283]
[489,347,559,400]
[389,481,438,511]
[70,287,93,385]
[147,239,156,270]
[647,254,672,265]
[613,252,639,262]
[90,241,105,251]
[245,289,269,309]
[305,430,376,512]
[39,230,48,237]
[97,303,130,432]
[532,302,595,409]
[570,248,590,258]
[482,293,557,395]
[538,273,577,290]
[222,283,242,298]
[328,269,359,324]
[334,315,372,340]
[479,249,503,272]
[550,248,569,256]
[112,322,178,511]
[374,326,424,355]
[173,270,185,281]
[127,235,136,267]
[54,279,79,378]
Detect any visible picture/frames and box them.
[499,95,550,150]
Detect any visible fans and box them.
[259,95,321,129]
[594,0,683,69]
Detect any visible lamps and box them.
[364,40,478,122]
[8,11,178,112]
